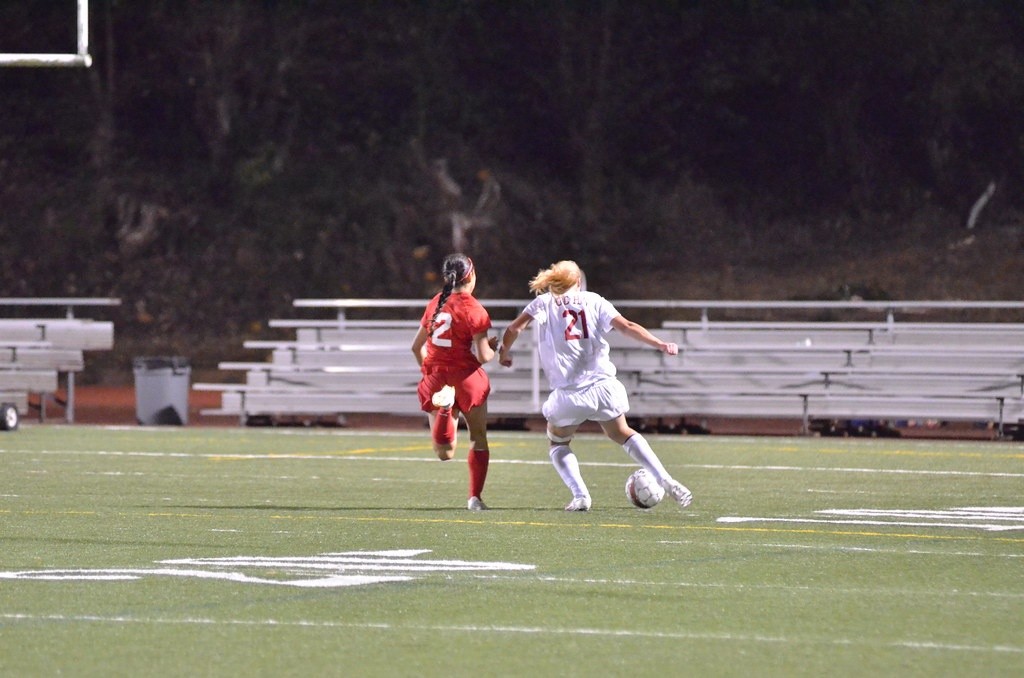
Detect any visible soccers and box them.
[625,469,665,508]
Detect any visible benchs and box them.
[0,289,1024,429]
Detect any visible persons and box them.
[499,261,692,511]
[412,252,500,510]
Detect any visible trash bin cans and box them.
[132,355,193,426]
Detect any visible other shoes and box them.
[565,497,591,510]
[468,496,488,510]
[672,487,693,508]
[432,384,456,409]
